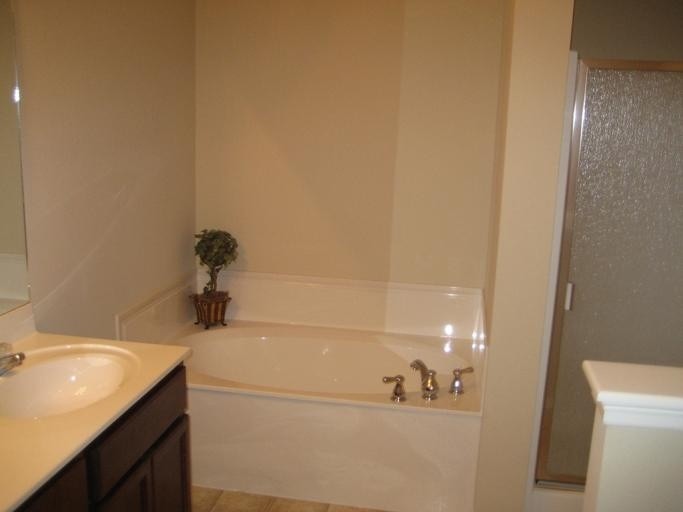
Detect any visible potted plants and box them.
[188,229,239,328]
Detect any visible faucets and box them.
[0,353,23,374]
[411,359,438,398]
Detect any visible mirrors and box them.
[0,0,32,316]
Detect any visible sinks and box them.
[0,351,128,416]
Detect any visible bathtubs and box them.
[176,320,481,512]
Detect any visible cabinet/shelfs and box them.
[13,363,192,512]
